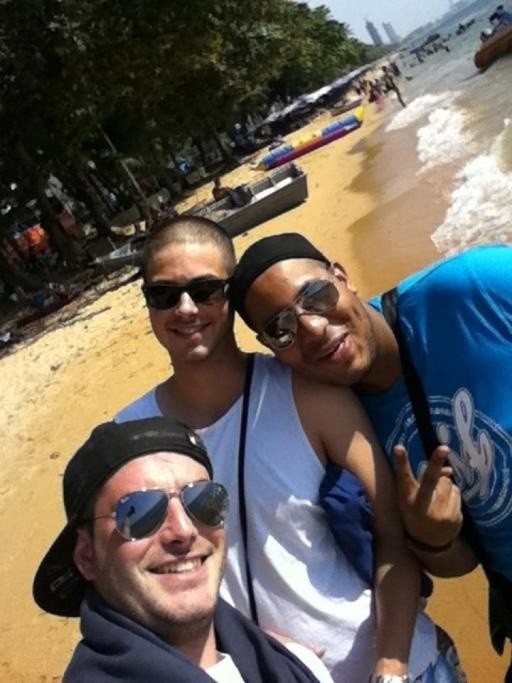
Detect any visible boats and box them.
[330,97,363,116]
[247,95,371,171]
[469,23,512,70]
[85,157,314,273]
[408,32,440,54]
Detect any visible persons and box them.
[111,215,471,683]
[33,415,332,683]
[226,232,512,683]
[128,3,511,244]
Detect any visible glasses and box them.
[91,479,232,542]
[257,279,341,351]
[139,277,228,310]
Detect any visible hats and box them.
[31,416,213,618]
[226,233,329,311]
[317,461,433,594]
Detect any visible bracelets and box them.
[403,529,453,553]
[366,674,407,682]
